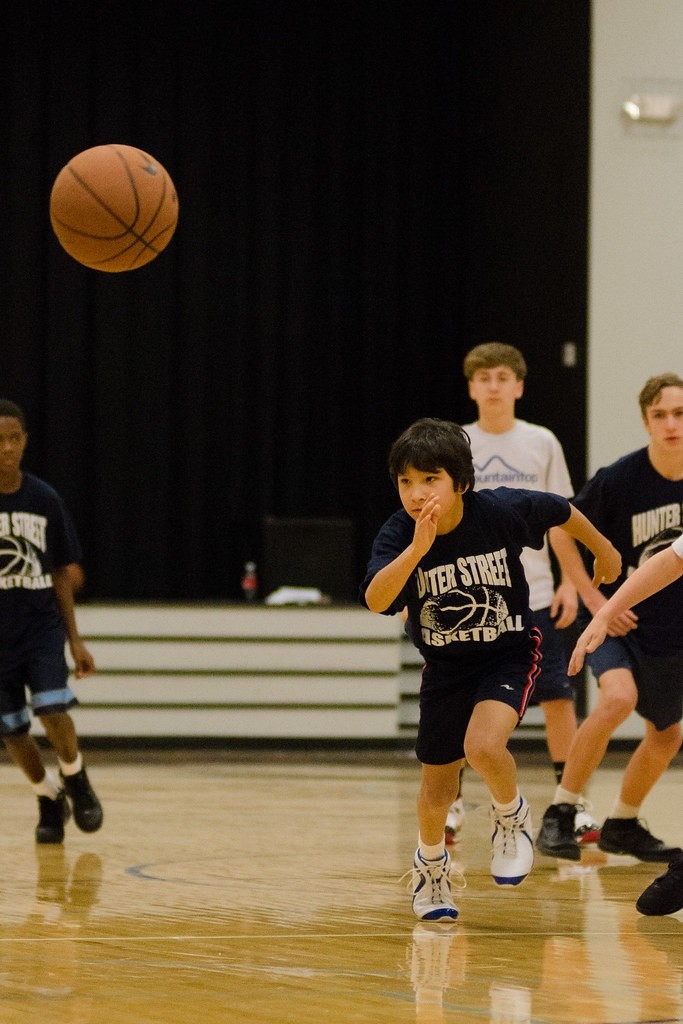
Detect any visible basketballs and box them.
[50,143,180,272]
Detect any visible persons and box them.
[358,417,623,923]
[401,343,605,845]
[534,371,683,917]
[0,397,104,843]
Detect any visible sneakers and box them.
[60,764,104,832]
[37,845,66,905]
[475,794,535,889]
[411,924,460,994]
[574,799,603,843]
[35,787,71,843]
[636,860,683,916]
[397,848,466,922]
[445,798,466,844]
[598,815,682,862]
[536,803,585,861]
[67,854,104,911]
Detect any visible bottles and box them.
[241,561,257,600]
